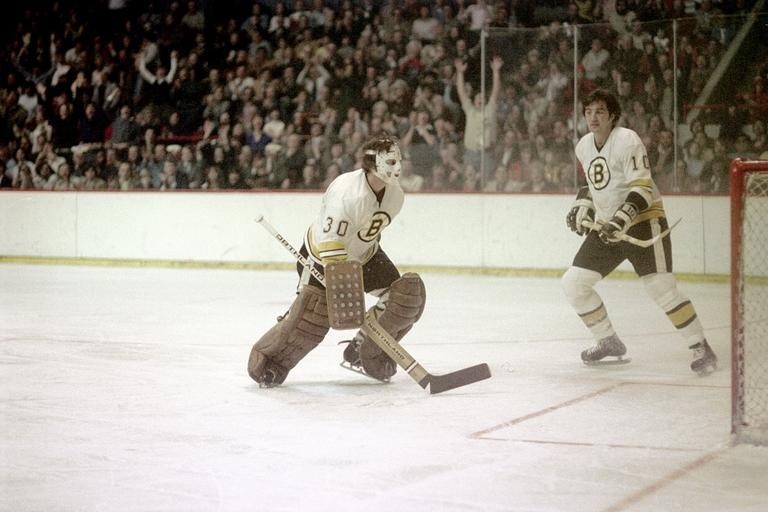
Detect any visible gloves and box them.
[596,188,653,249]
[564,183,593,236]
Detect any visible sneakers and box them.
[343,337,363,368]
[689,339,719,373]
[580,334,627,362]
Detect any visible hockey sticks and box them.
[254,213,492,394]
[581,213,684,247]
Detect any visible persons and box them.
[245,134,426,386]
[560,87,716,370]
[0,0,767,199]
